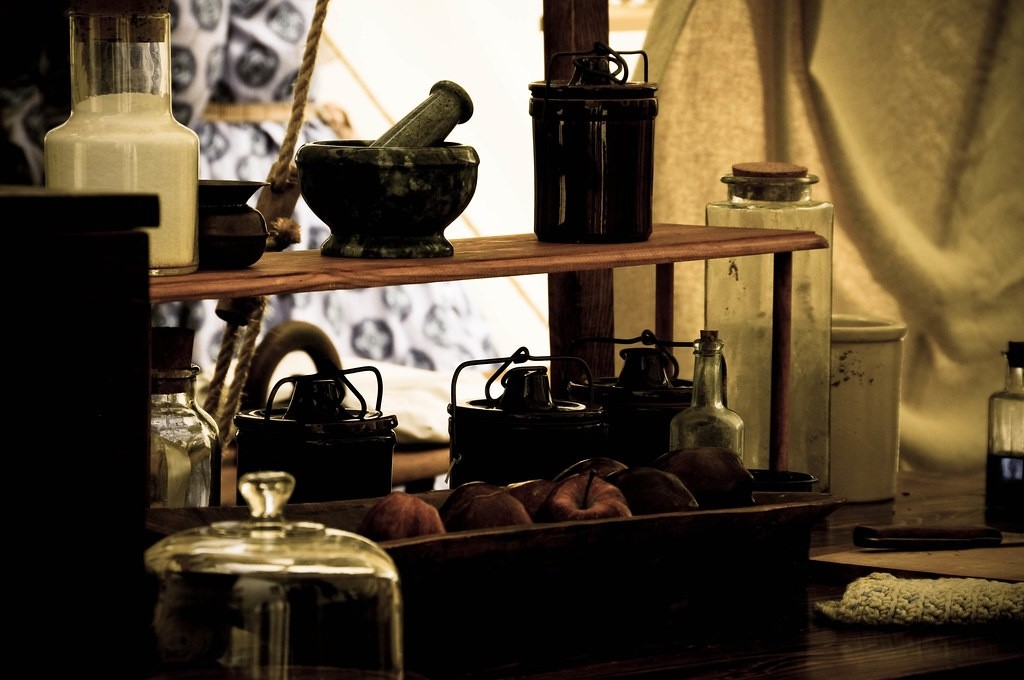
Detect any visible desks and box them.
[420,471,1024,680]
[148,221,829,471]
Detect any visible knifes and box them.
[851,522,1023,550]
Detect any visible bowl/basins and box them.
[295,138,481,261]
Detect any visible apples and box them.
[359,449,754,546]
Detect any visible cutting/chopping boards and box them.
[809,537,1024,587]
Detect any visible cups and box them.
[709,305,909,507]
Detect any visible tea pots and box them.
[144,466,403,680]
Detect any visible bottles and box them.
[983,340,1023,533]
[41,0,201,277]
[667,327,744,466]
[144,356,221,509]
[699,162,834,496]
